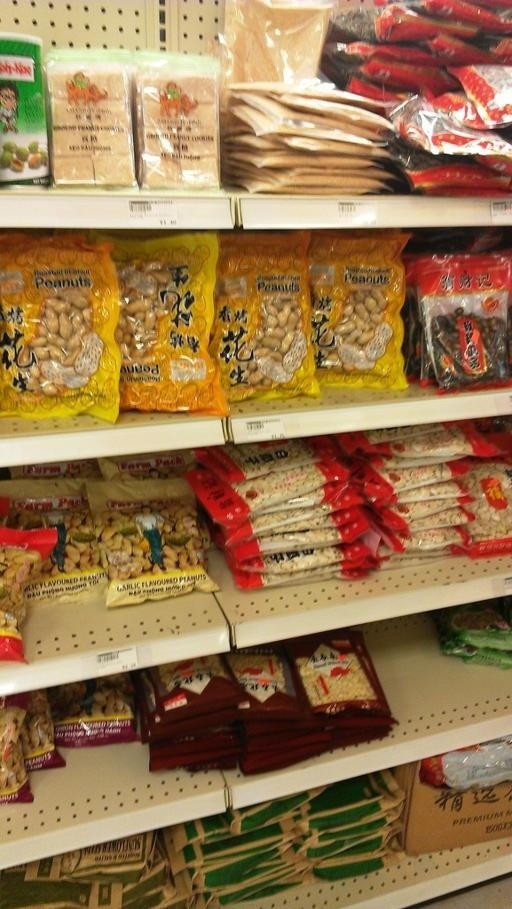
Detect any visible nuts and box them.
[1,232,406,415]
[402,303,511,392]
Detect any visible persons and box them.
[39,508,74,576]
[133,504,172,574]
[148,467,161,478]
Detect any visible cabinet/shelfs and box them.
[1,194,511,909]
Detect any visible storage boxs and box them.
[392,754,511,852]
